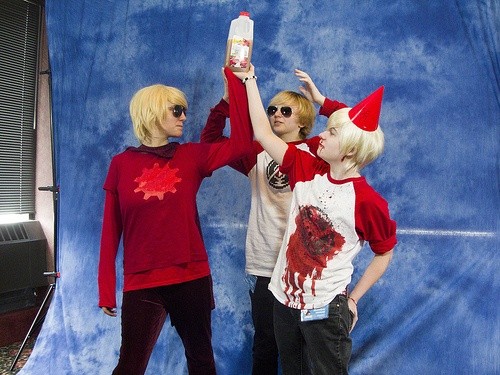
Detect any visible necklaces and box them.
[242,75,257,84]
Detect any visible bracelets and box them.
[349,296,358,305]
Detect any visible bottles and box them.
[224,12,255,72]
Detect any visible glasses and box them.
[266,105,293,118]
[168,105,187,118]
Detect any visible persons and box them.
[98,61,254,375]
[232,61,397,375]
[200,66,351,375]
